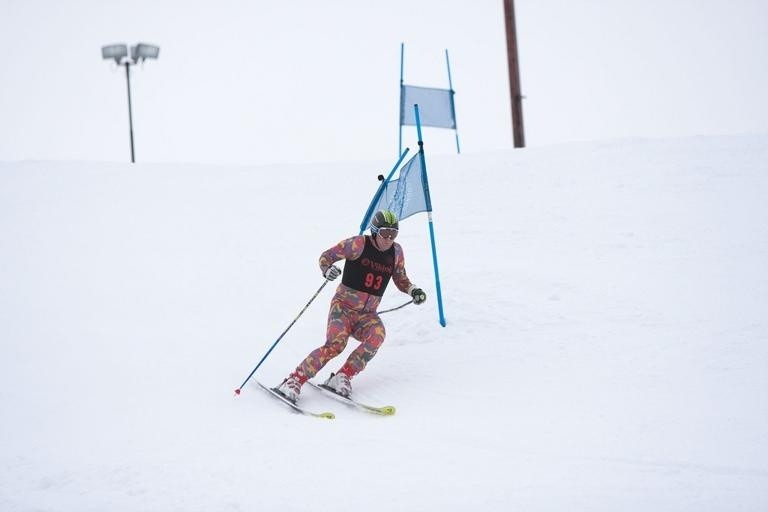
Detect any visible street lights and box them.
[102,44,159,162]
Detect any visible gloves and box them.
[325,265,341,281]
[411,288,427,305]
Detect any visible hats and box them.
[370,210,399,238]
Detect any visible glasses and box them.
[369,222,399,240]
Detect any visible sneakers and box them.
[329,373,353,396]
[279,378,301,402]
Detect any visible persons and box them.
[271,209,427,405]
